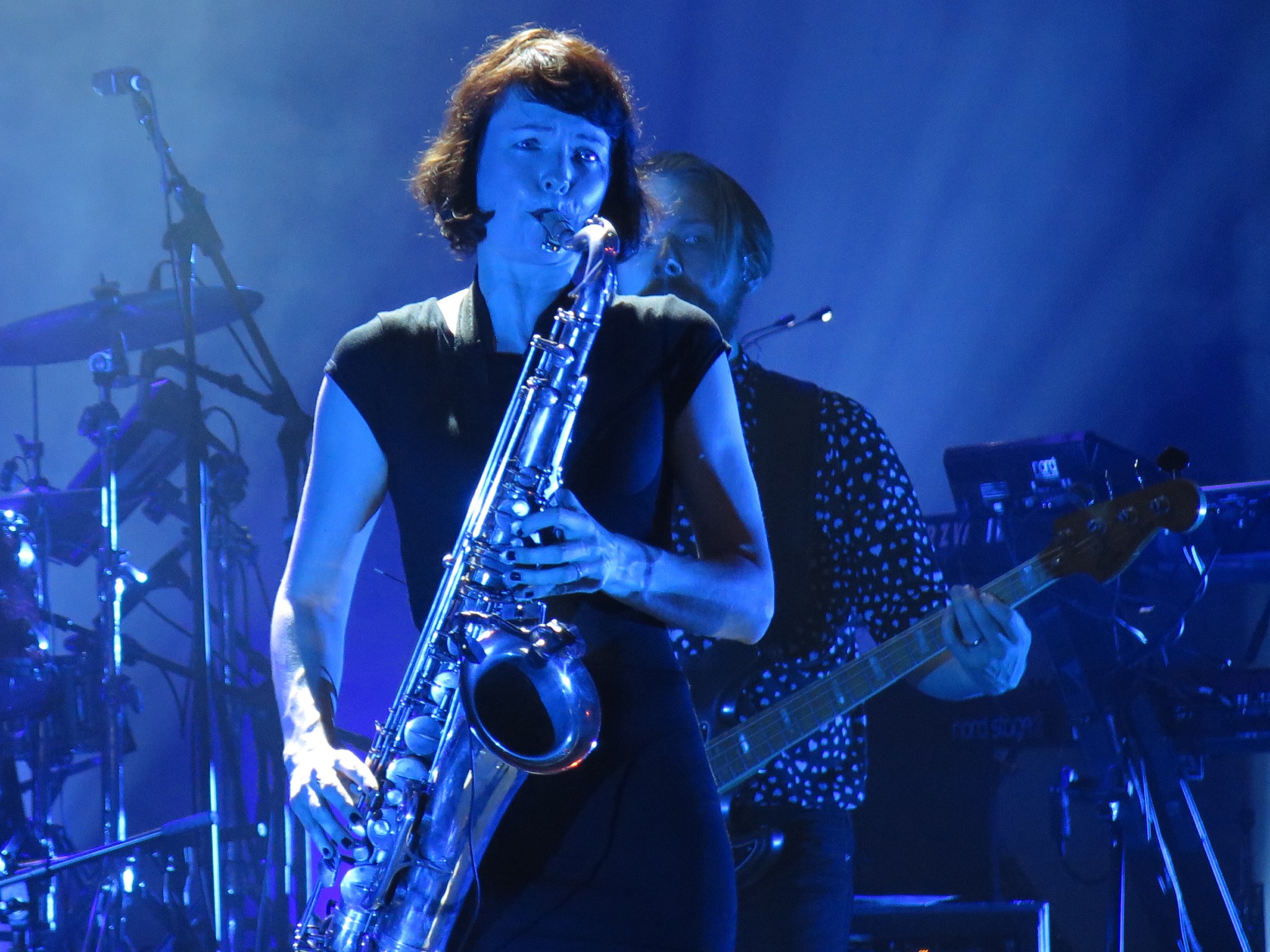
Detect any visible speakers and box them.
[852,477,1270,952]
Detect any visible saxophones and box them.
[292,213,619,952]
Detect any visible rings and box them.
[964,635,983,648]
[573,561,582,581]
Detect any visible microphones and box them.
[136,271,161,401]
[525,622,576,671]
[91,69,148,96]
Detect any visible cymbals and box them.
[0,285,263,367]
[0,487,100,505]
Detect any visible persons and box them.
[270,27,774,952]
[612,151,1031,952]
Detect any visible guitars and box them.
[703,447,1208,797]
